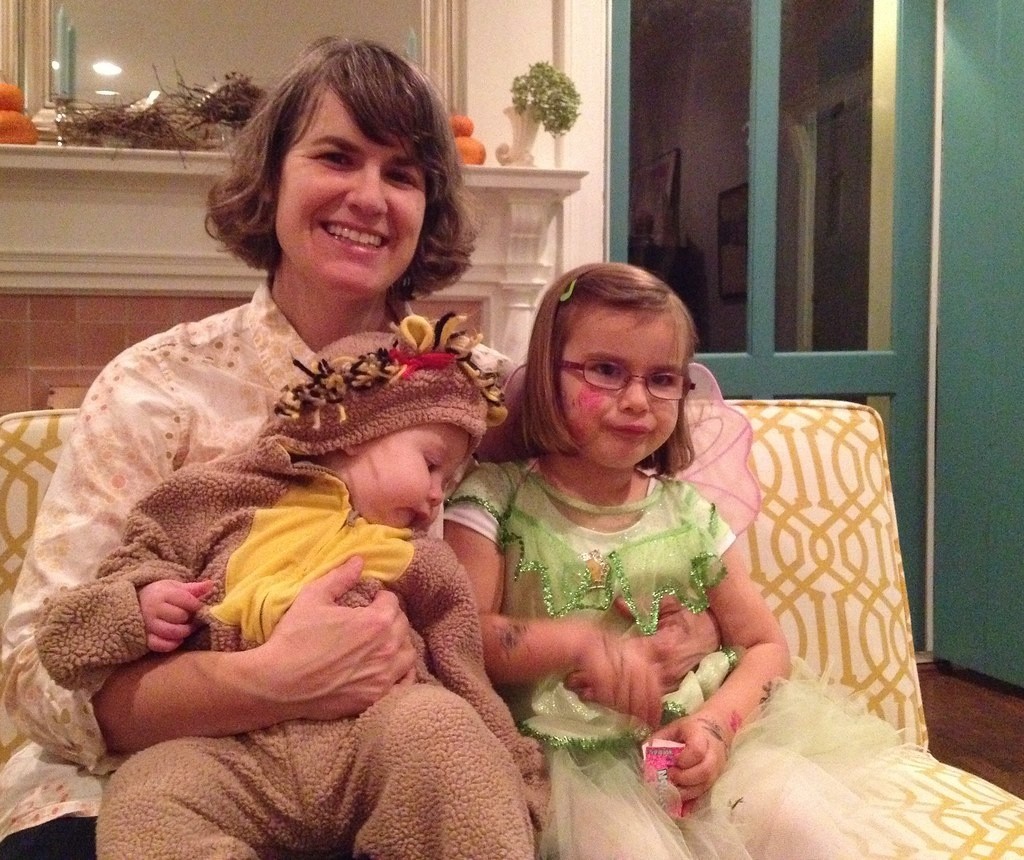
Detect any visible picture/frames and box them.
[716,185,747,302]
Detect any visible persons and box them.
[442,263,900,860]
[0,29,720,860]
[35,312,549,860]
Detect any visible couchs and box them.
[0,399,1024,859]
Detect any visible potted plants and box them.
[494,61,581,166]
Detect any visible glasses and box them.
[554,359,696,399]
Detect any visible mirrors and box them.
[7,0,468,144]
